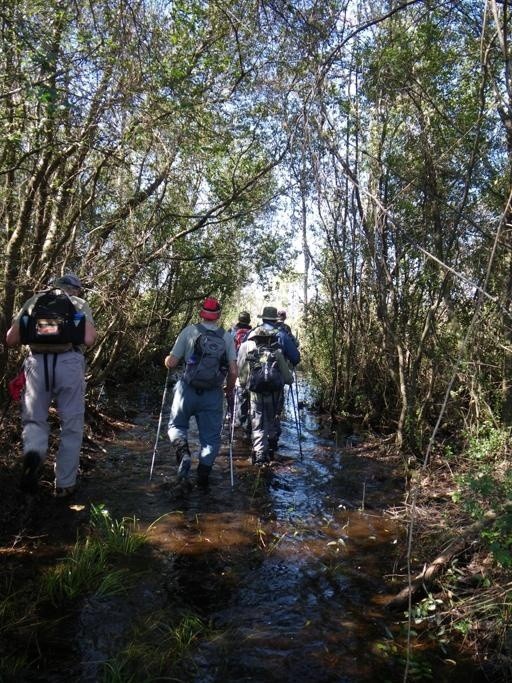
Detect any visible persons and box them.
[6,275,97,498]
[225,307,300,466]
[165,298,238,489]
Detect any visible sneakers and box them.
[53,480,82,498]
[198,462,212,472]
[23,451,41,501]
[177,452,192,479]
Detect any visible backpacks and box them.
[248,326,284,394]
[20,288,85,355]
[233,323,251,348]
[182,322,228,388]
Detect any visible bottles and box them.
[188,354,197,365]
[73,312,84,326]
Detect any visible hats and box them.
[278,311,286,319]
[239,312,250,325]
[200,298,221,320]
[55,273,82,289]
[257,306,281,321]
